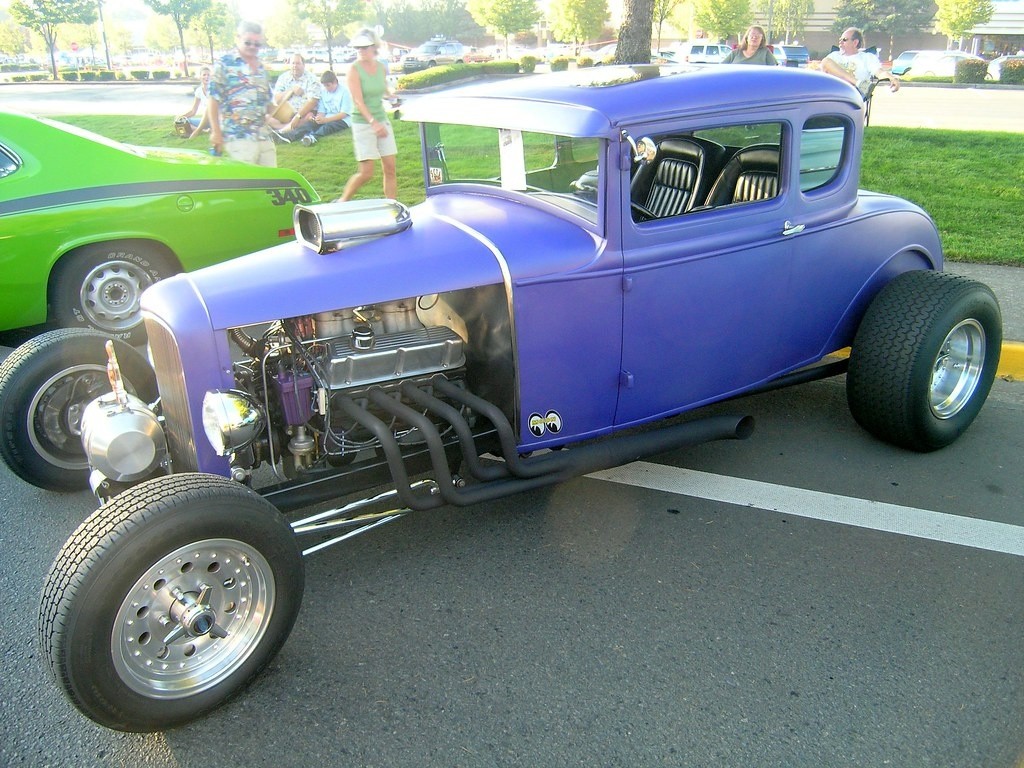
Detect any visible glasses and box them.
[839,37,854,41]
[240,37,263,47]
[353,45,371,50]
[749,32,763,38]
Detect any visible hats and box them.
[347,35,374,46]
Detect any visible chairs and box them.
[702,142,784,212]
[625,135,727,224]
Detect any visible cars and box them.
[0,104,327,346]
[0,60,1008,737]
[580,40,1023,85]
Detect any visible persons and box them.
[332,29,401,202]
[265,51,322,132]
[173,65,213,137]
[719,25,779,65]
[822,27,899,99]
[273,72,353,145]
[208,24,284,170]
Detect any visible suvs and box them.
[403,33,470,71]
